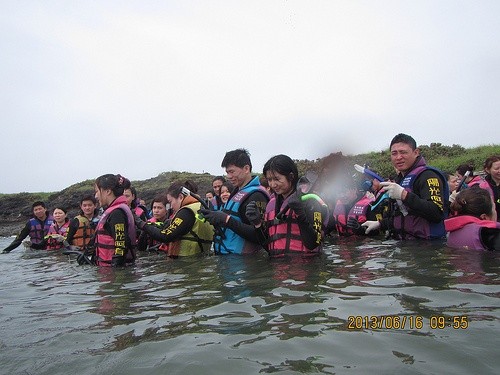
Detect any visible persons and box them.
[93,173,135,267]
[360,133,449,241]
[198,148,270,253]
[298,173,400,236]
[0,196,99,254]
[259,175,273,195]
[444,154,500,251]
[124,176,234,256]
[246,155,329,252]
